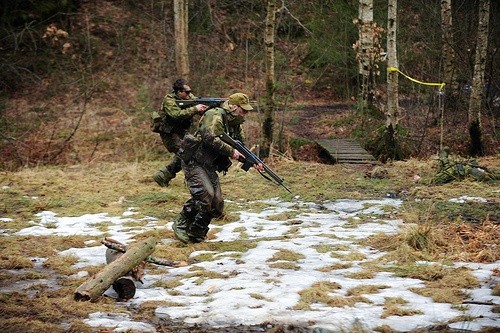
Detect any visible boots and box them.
[171,214,189,243]
[154,167,173,187]
[187,224,205,242]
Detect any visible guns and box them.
[175,96,257,116]
[219,131,292,194]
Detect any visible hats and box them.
[229,93,254,111]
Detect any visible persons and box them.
[150,79,210,188]
[171,92,266,245]
